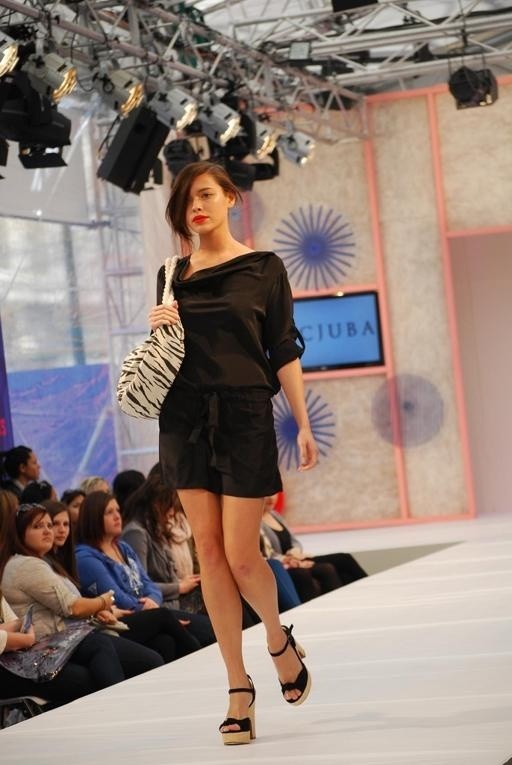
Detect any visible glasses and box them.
[17,503,47,516]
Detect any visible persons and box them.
[1,447,217,705]
[260,490,367,614]
[149,162,321,745]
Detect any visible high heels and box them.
[219,674,256,744]
[268,624,311,706]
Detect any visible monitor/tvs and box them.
[267,290,385,373]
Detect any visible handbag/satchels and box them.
[116,317,186,421]
[0,618,97,684]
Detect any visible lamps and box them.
[0,29,316,197]
[446,26,499,109]
